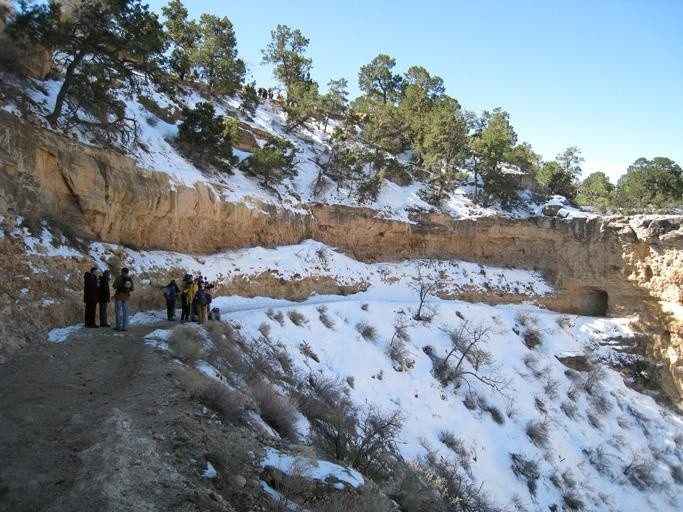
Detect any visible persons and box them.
[163,271,214,324]
[82,267,100,329]
[97,270,111,327]
[110,267,134,331]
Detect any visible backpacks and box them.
[199,294,207,305]
[181,293,188,305]
[117,276,133,293]
